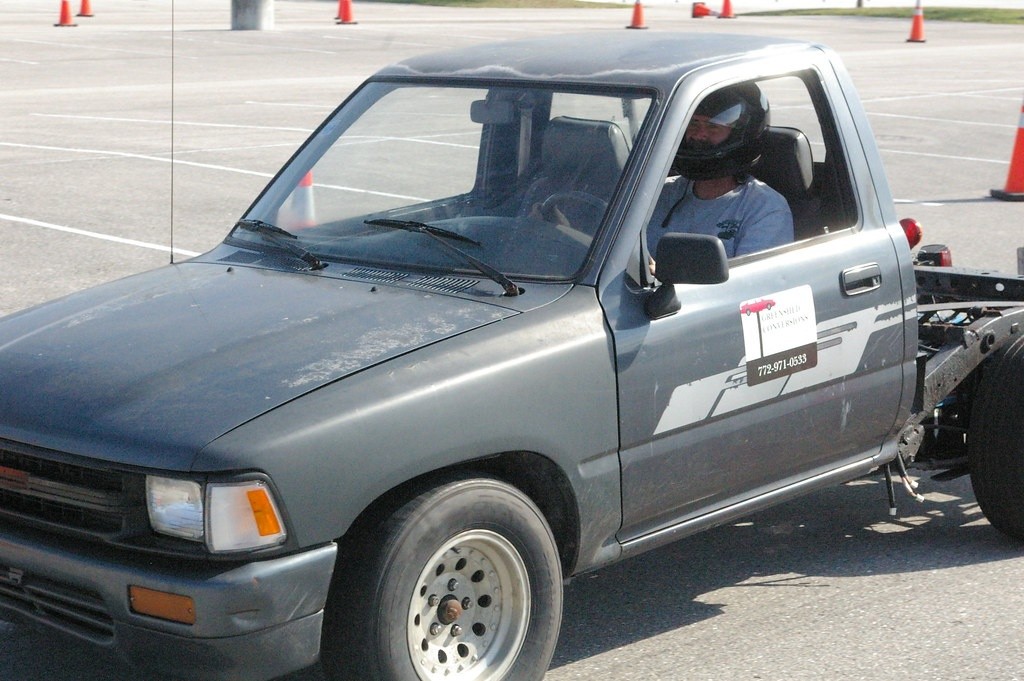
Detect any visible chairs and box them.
[751,125,823,241]
[485,115,630,235]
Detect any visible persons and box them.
[526,77,797,259]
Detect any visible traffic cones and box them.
[53,0,79,27]
[334,1,358,24]
[989,105,1024,202]
[288,168,315,229]
[76,0,95,17]
[906,0,926,42]
[691,2,720,18]
[626,0,649,29]
[717,0,737,19]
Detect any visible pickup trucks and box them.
[1,30,1024,681]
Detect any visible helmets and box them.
[672,83,771,184]
